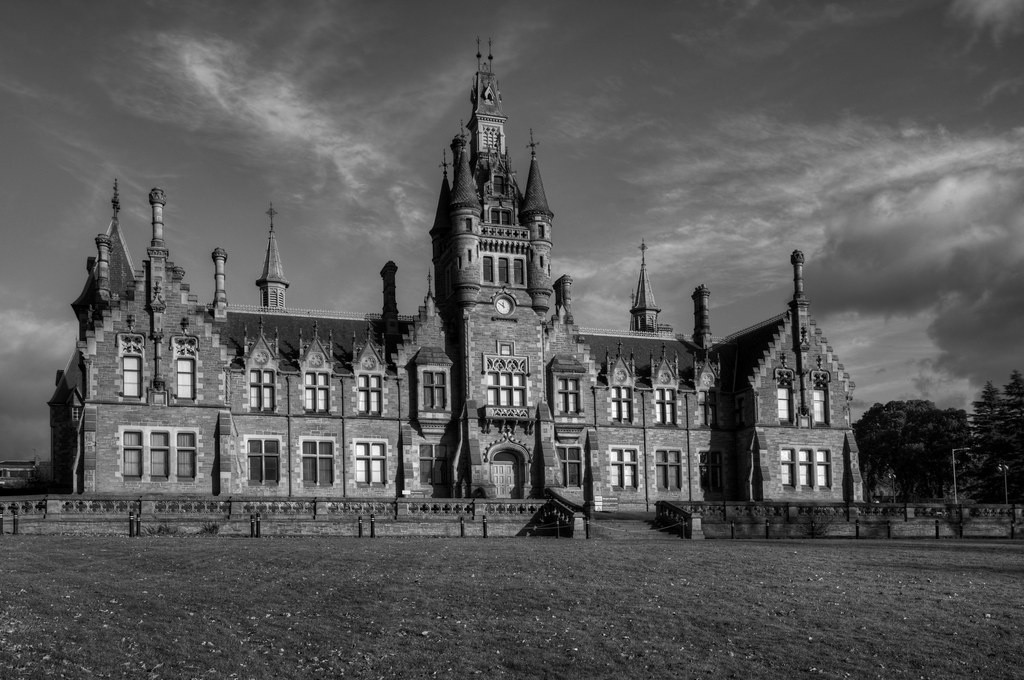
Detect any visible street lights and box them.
[952,447,971,504]
[888,473,896,503]
[997,459,1010,505]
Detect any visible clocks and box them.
[496,298,511,315]
